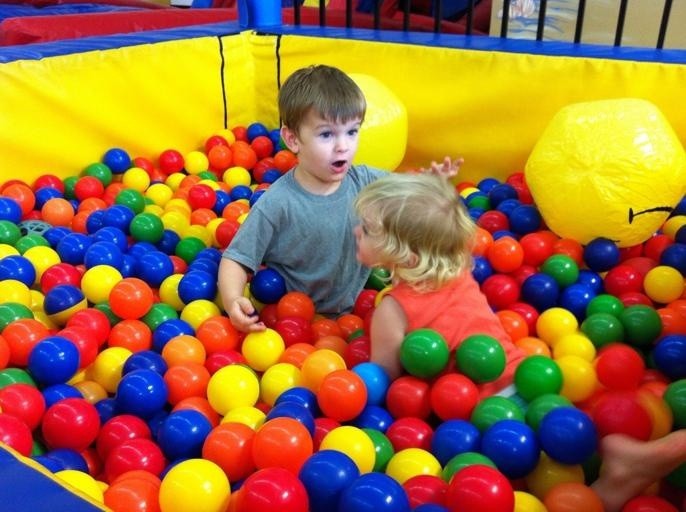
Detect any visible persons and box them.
[352,175,524,403]
[217,64,464,335]
[595,429,685,512]
